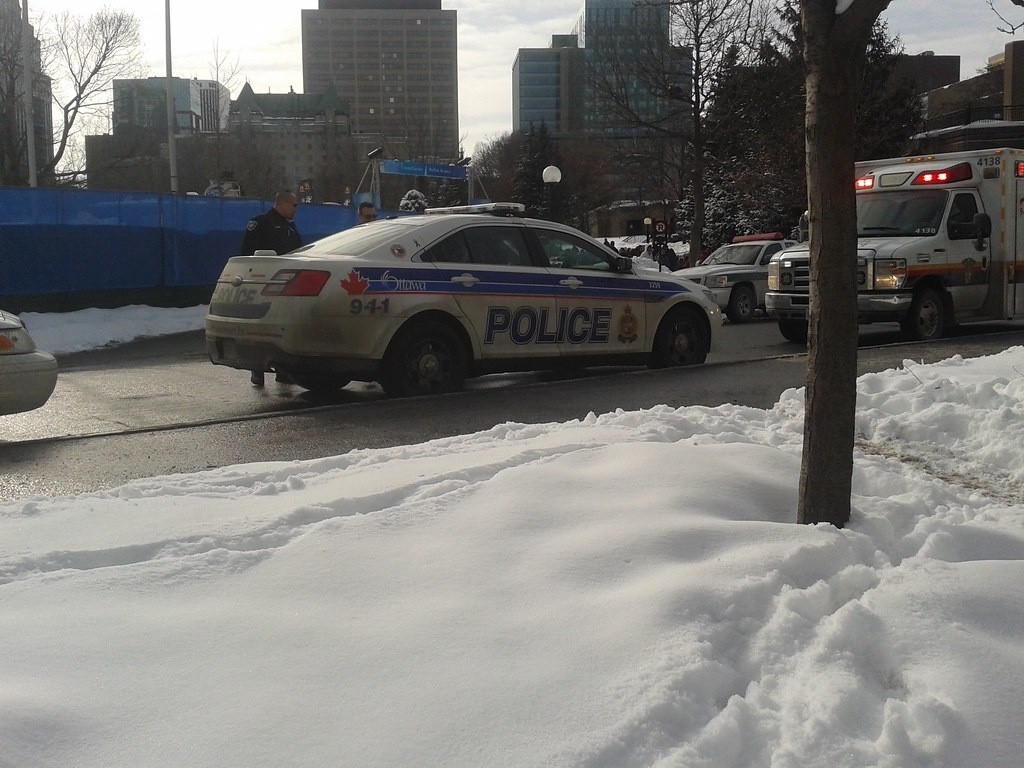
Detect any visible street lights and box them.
[541,166,562,223]
[644,217,652,244]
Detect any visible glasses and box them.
[360,214,378,219]
[286,201,298,208]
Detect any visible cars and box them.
[205,201,724,398]
[664,233,801,323]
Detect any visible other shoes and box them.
[251,368,264,384]
[276,372,294,384]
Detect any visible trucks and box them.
[763,147,1024,344]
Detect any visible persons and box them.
[639,244,654,260]
[358,202,379,226]
[240,189,306,387]
[572,238,645,260]
